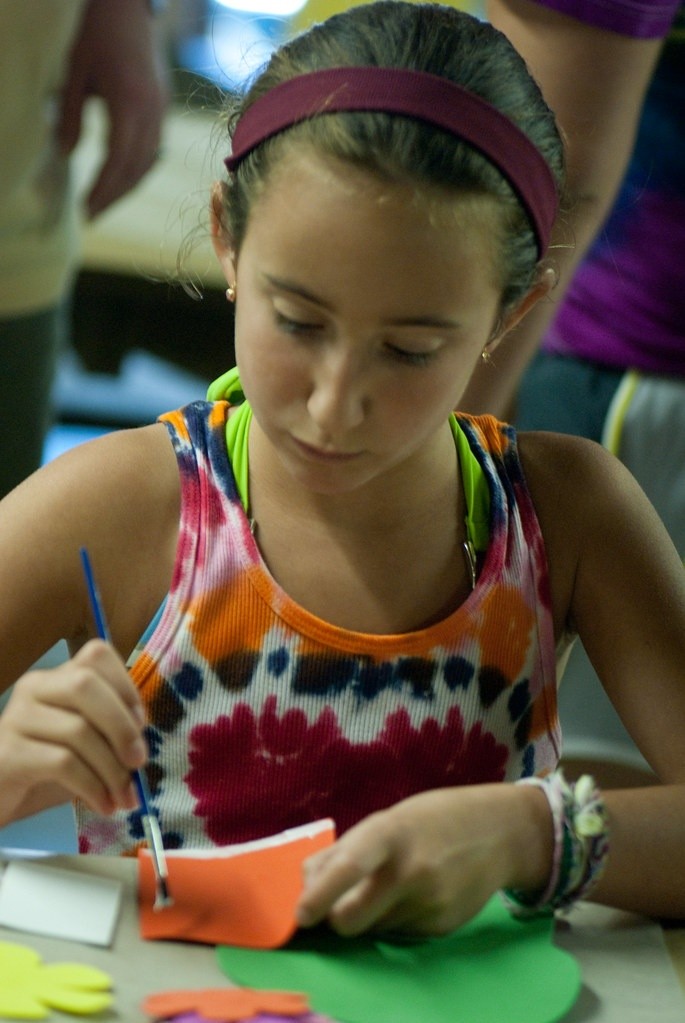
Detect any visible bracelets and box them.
[503,771,605,921]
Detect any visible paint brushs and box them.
[80,547,175,908]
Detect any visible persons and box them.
[484,1,685,436]
[0,0,160,499]
[0,0,685,941]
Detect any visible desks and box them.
[0,853,685,1023]
